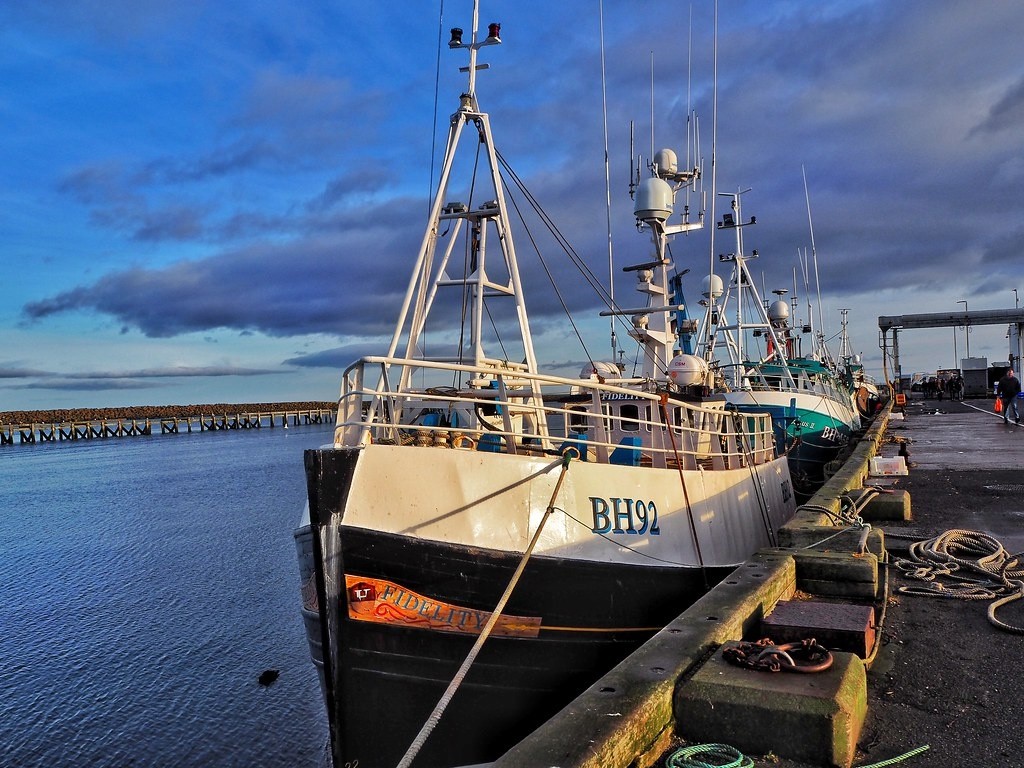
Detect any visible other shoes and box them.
[1005,419,1022,425]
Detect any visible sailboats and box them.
[800,166,880,422]
[293,0,797,768]
[689,0,862,460]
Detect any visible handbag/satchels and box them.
[994,398,1001,413]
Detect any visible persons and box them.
[996,368,1022,424]
[921,376,964,402]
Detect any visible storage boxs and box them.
[888,412,904,419]
[870,456,909,476]
[896,394,905,404]
